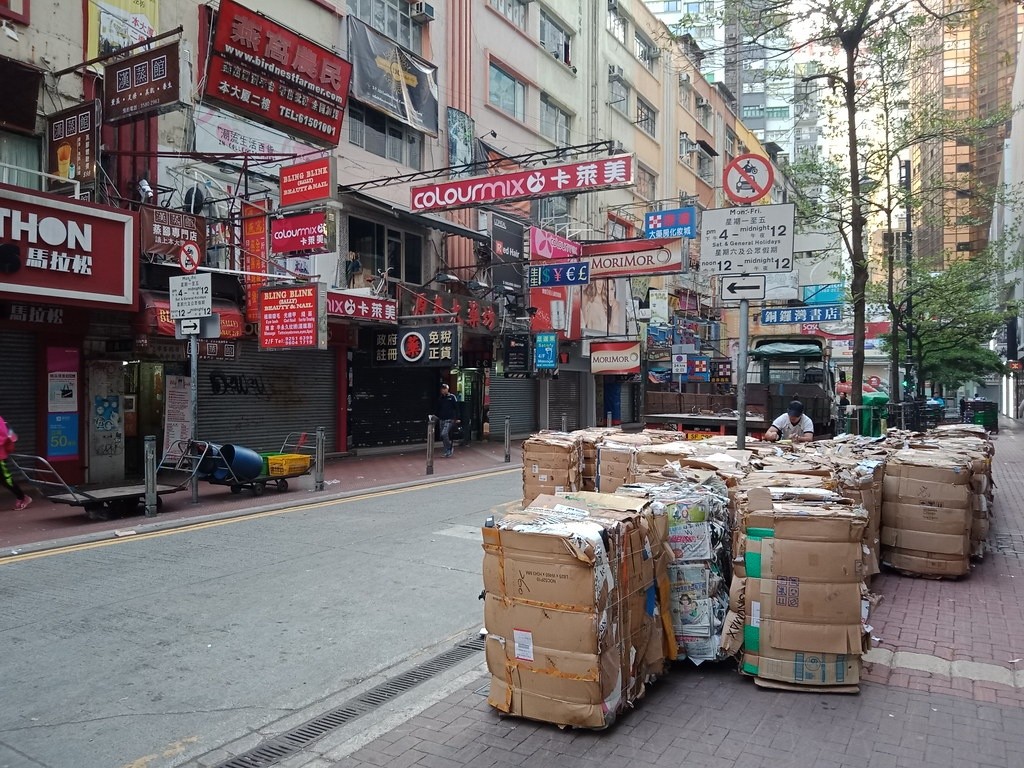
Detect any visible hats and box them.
[439,383,449,389]
[787,401,803,416]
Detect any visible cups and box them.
[56,147,72,182]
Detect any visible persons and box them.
[432,383,461,458]
[959,395,967,423]
[607,278,625,334]
[836,392,850,406]
[582,280,607,334]
[450,122,472,172]
[931,391,945,408]
[765,401,814,443]
[0,416,32,512]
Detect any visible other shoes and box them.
[447,447,454,457]
[441,449,448,457]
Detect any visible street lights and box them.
[859,148,913,402]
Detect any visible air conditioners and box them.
[412,1,435,24]
[682,73,690,83]
[609,65,623,81]
[698,98,709,107]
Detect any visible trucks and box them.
[640,332,840,443]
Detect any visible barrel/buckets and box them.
[213,443,262,481]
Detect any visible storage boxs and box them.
[680,393,712,413]
[645,390,680,414]
[712,396,737,412]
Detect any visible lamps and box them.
[455,282,488,293]
[423,274,459,289]
[479,285,514,299]
[480,130,497,138]
[533,158,547,165]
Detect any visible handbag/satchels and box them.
[4,429,18,452]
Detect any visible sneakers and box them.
[14,495,32,510]
